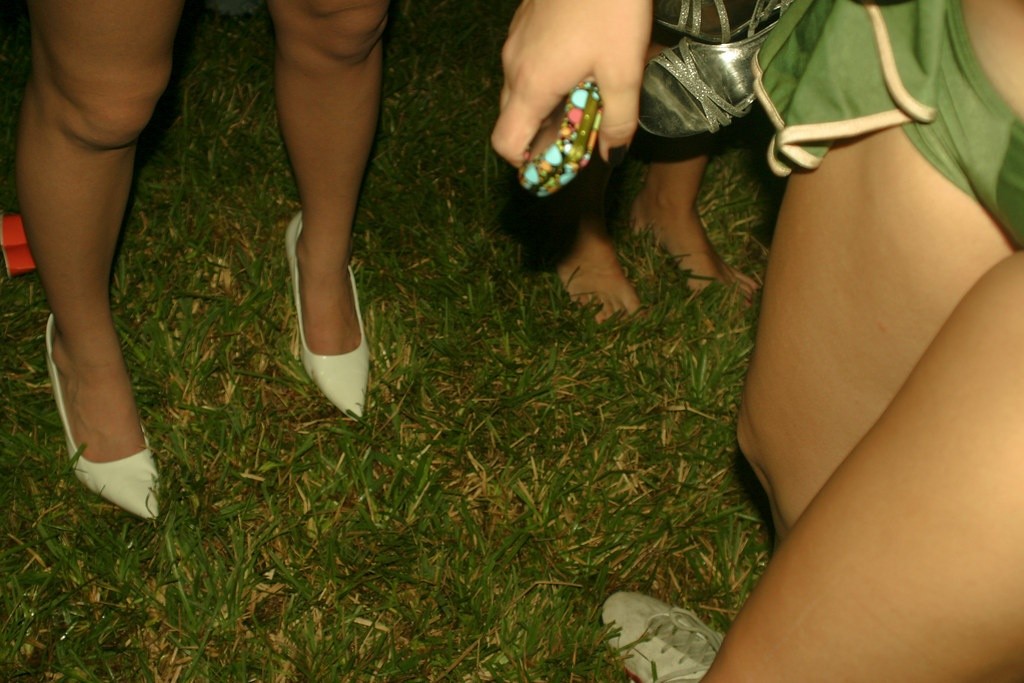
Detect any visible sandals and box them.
[636,0,798,138]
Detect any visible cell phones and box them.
[517,81,604,198]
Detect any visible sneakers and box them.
[602,591,722,682]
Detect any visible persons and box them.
[556,28,759,325]
[17,0,388,521]
[491,0,1024,683]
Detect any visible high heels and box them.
[44,312,160,522]
[287,210,370,422]
